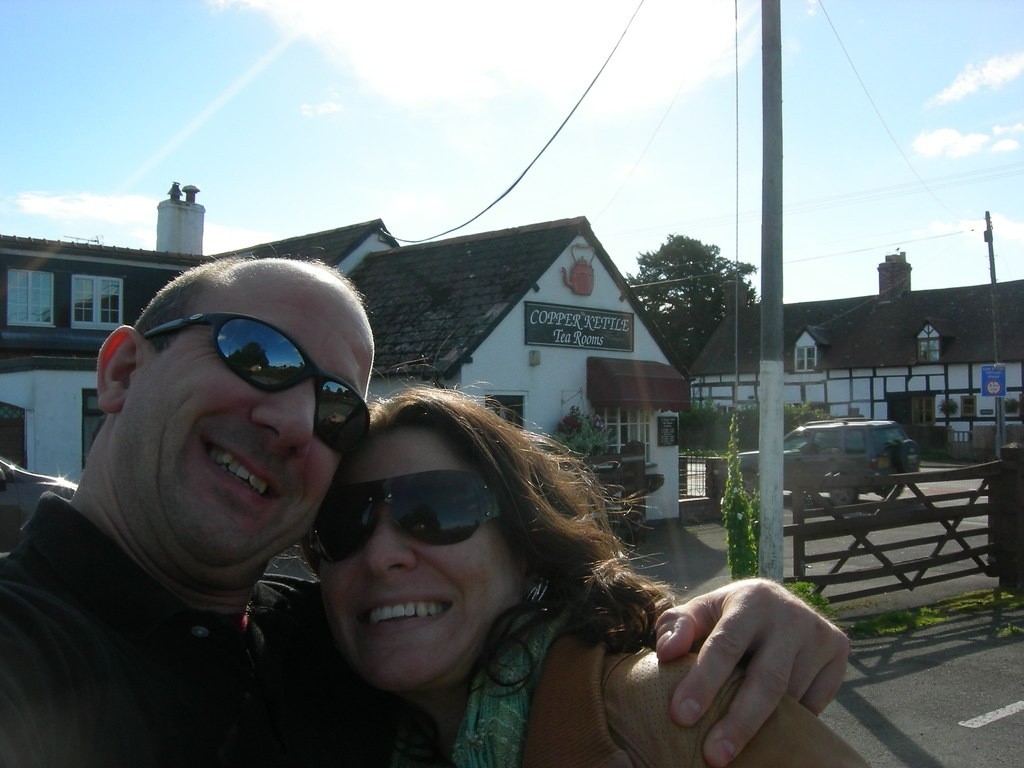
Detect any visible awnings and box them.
[586,356,693,415]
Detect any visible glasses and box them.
[142,311,371,452]
[312,470,503,565]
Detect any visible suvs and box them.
[737,417,919,505]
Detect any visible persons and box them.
[298,388,875,768]
[1,256,851,768]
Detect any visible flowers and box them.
[558,404,607,437]
